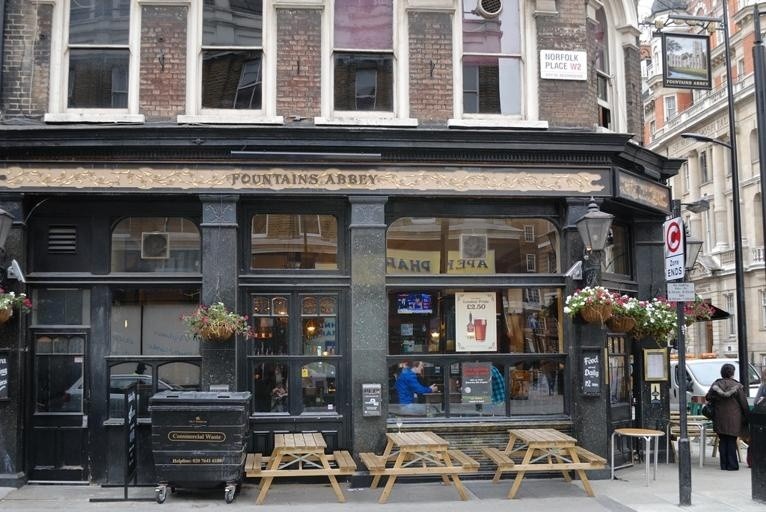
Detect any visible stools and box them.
[663,406,745,468]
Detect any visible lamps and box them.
[682,234,704,270]
[0,208,15,260]
[575,193,616,254]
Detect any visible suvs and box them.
[61,371,176,416]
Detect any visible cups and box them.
[474,318,488,342]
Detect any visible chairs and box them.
[388,386,420,417]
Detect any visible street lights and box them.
[679,130,751,400]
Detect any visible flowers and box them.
[0,287,32,315]
[181,302,253,344]
[561,285,715,349]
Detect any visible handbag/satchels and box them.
[701,403,714,419]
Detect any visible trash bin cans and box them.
[147,391,253,504]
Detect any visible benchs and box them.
[570,444,607,470]
[357,449,394,477]
[326,449,357,476]
[482,447,520,472]
[445,449,479,473]
[244,453,269,479]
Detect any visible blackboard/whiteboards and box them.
[0,354,11,402]
[578,346,603,398]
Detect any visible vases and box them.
[200,325,231,344]
[0,308,12,324]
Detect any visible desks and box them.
[609,428,665,486]
[422,391,462,417]
[254,432,345,506]
[490,428,595,499]
[372,432,470,503]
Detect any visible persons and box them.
[509,360,525,371]
[745,408,755,468]
[398,296,409,309]
[528,366,551,401]
[414,293,422,308]
[255,360,288,412]
[392,358,423,402]
[476,359,507,415]
[755,368,766,406]
[395,358,438,415]
[705,363,750,471]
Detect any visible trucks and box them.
[670,352,763,414]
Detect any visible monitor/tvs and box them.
[394,291,435,316]
